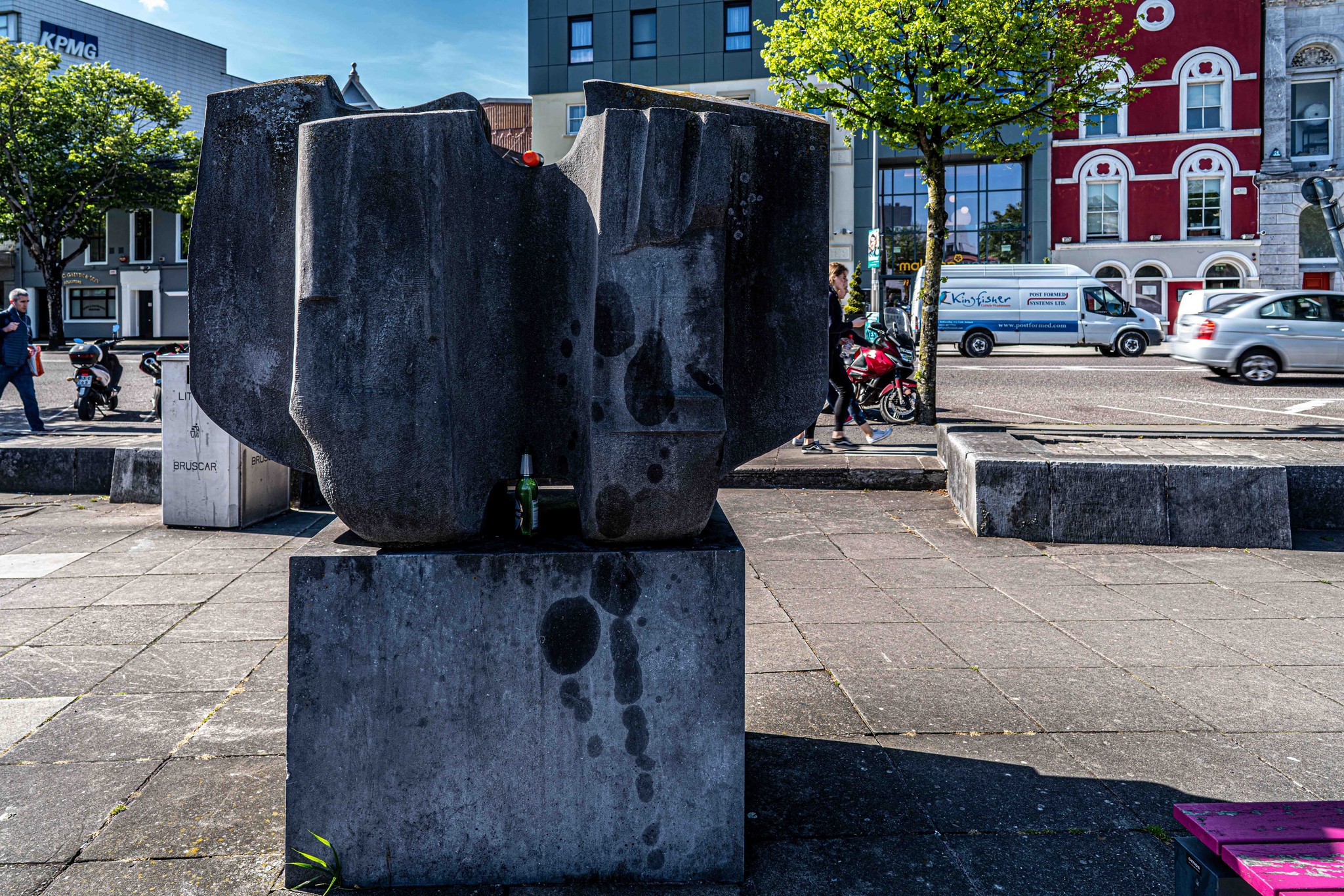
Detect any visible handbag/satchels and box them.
[26,344,44,377]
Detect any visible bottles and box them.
[514,450,539,537]
[522,150,545,167]
[840,335,854,360]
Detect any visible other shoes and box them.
[43,428,59,432]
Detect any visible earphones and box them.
[12,302,15,305]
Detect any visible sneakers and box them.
[865,428,892,444]
[792,435,819,447]
[801,442,833,454]
[829,437,859,450]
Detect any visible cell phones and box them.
[862,313,872,319]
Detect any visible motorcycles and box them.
[819,307,919,427]
[138,342,190,421]
[67,325,125,421]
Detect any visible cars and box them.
[1167,288,1344,387]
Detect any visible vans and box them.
[909,263,1165,357]
[1174,288,1323,336]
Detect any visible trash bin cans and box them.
[1171,799,1344,896]
[156,352,291,531]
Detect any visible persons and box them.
[0,288,57,433]
[792,263,893,455]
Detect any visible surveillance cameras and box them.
[1260,230,1266,235]
